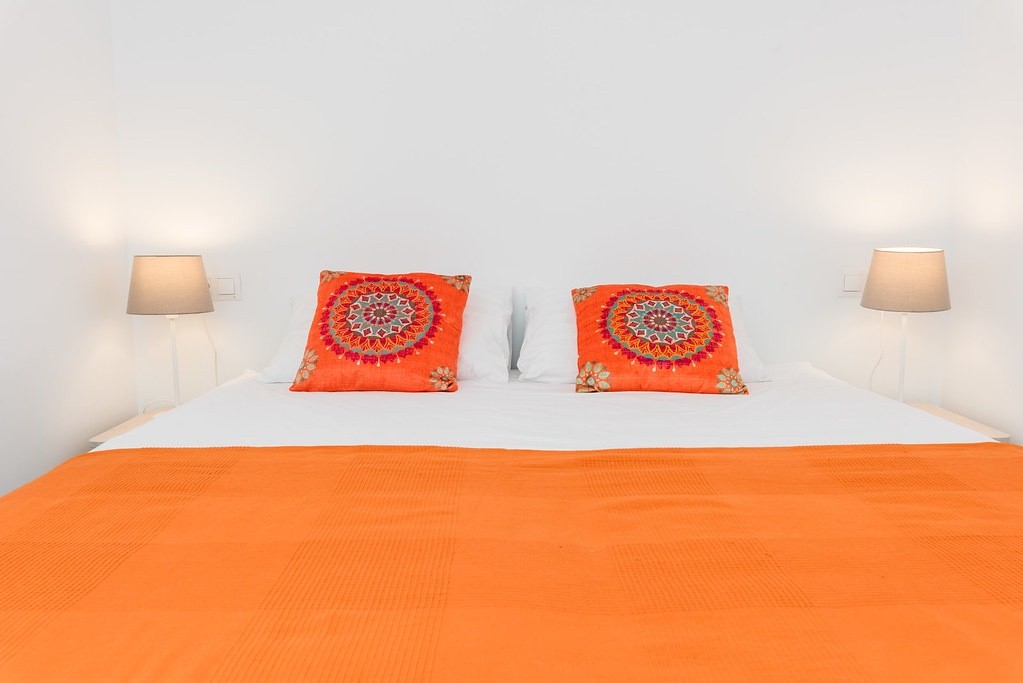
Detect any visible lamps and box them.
[125,255,216,402]
[859,247,951,403]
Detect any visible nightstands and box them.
[896,397,1011,442]
[89,403,176,443]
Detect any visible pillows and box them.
[289,270,473,392]
[517,294,771,382]
[569,285,750,395]
[258,289,510,384]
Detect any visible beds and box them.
[0,361,1023,682]
